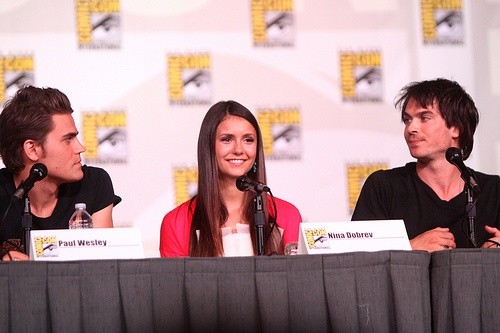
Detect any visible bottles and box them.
[69,204,93,229]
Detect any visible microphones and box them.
[446,147,480,193]
[236,175,270,192]
[11,163,47,203]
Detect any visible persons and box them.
[159,100,302,257]
[350,78,500,251]
[0,84,122,262]
